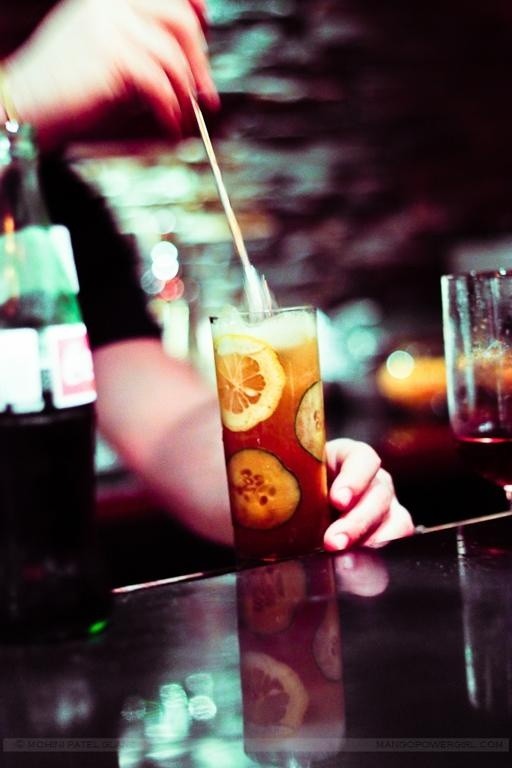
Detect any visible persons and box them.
[1,0,414,611]
[331,550,391,599]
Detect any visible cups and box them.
[208,303,329,572]
[437,268,512,446]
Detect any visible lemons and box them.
[295,380,327,465]
[225,445,300,533]
[215,335,286,434]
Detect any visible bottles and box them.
[0,120,99,586]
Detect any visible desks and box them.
[3,516,512,767]
